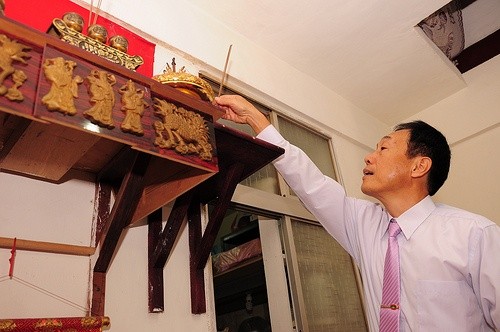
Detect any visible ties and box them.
[378,219,402,332]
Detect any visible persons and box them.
[119,79,148,136]
[42,56,84,116]
[212,92,500,332]
[83,69,118,129]
